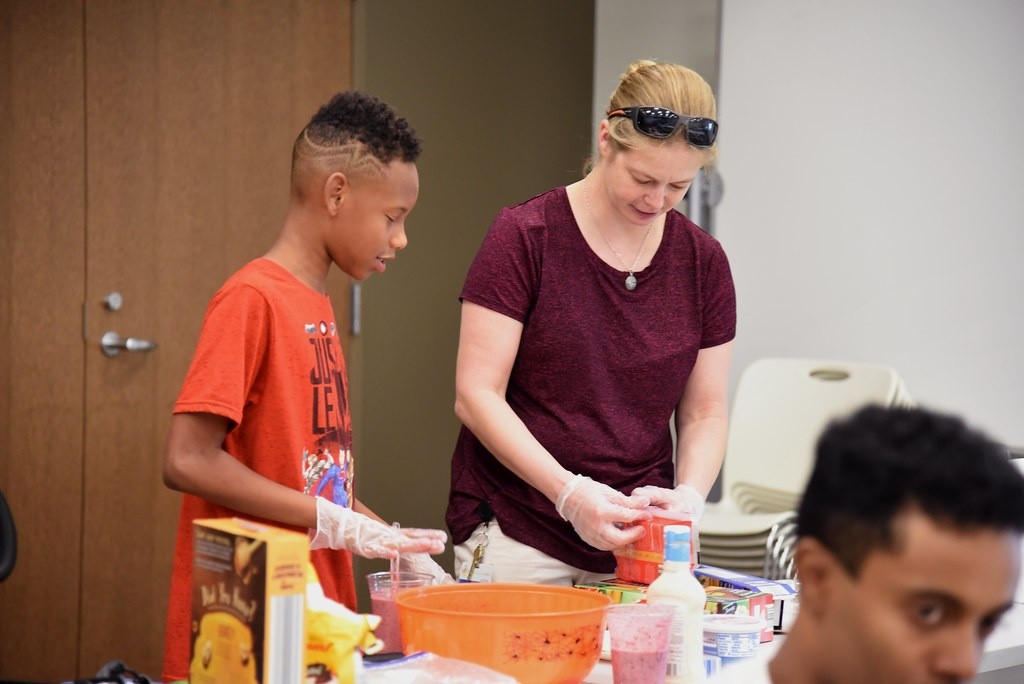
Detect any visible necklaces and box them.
[582,177,655,290]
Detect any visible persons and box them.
[705,398,1024,684]
[163,89,447,684]
[444,60,736,590]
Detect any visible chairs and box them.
[697,359,919,581]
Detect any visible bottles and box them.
[645,524,706,684]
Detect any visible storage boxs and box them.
[614,517,694,584]
[190,518,310,683]
[575,564,798,643]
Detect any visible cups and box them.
[367,570,435,655]
[603,602,674,684]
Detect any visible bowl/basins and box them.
[394,582,611,684]
[702,613,762,678]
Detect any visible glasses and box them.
[608,105,720,149]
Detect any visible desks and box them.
[584,600,1024,684]
